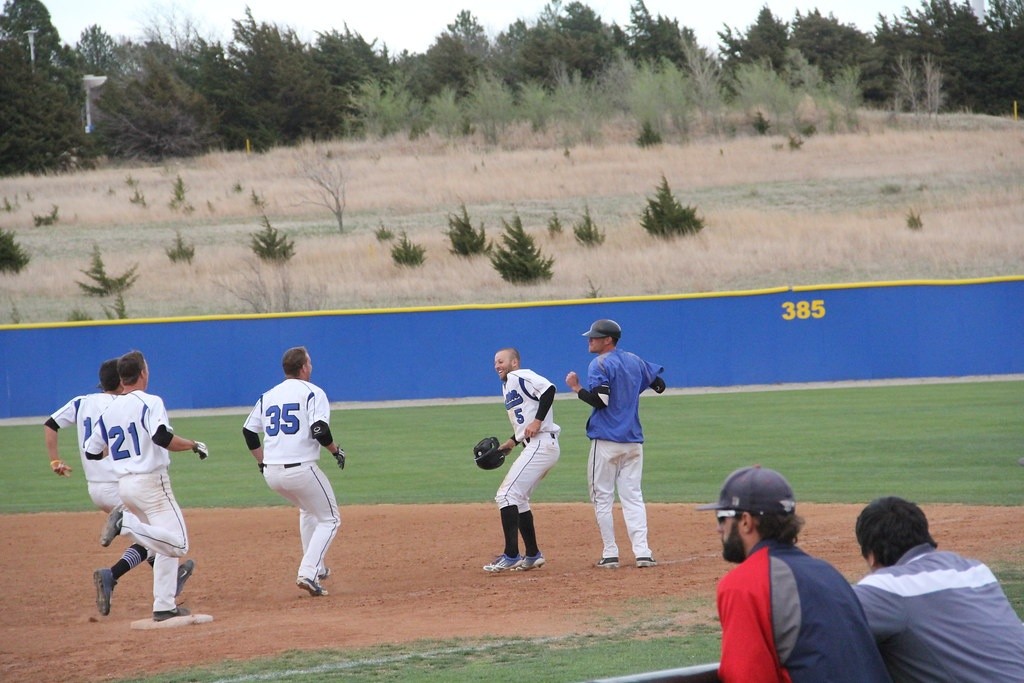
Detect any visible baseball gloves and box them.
[473,437,505,471]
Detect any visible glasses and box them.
[716,510,769,525]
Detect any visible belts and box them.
[284,462,302,469]
[523,433,557,447]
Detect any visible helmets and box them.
[582,319,621,341]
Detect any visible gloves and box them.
[193,441,208,460]
[332,445,345,469]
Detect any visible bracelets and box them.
[50,460,60,465]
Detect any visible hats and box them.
[694,467,796,517]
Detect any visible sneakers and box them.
[320,568,330,580]
[93,568,116,617]
[100,510,124,548]
[176,558,194,597]
[636,555,657,567]
[596,557,619,568]
[511,551,544,572]
[482,551,521,572]
[152,606,189,622]
[295,574,327,596]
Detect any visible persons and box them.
[695,465,893,683]
[850,496,1024,683]
[242,346,345,596]
[42,358,193,598]
[85,351,208,621]
[483,348,561,573]
[566,320,666,567]
